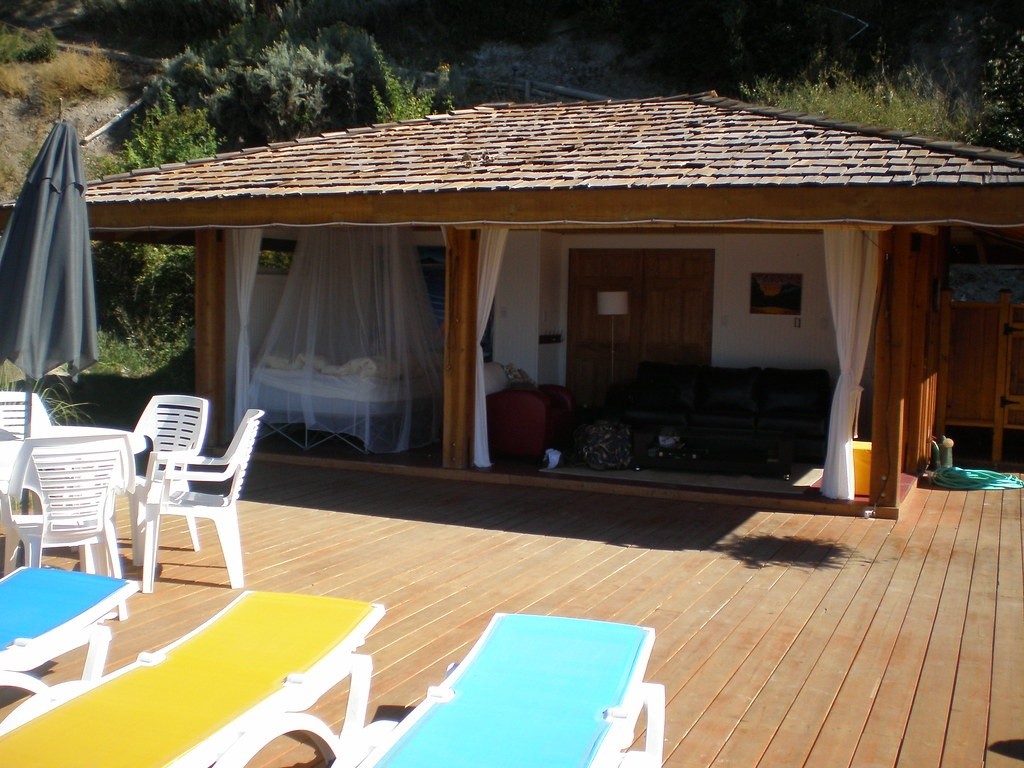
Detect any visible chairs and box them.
[111,396,265,593]
[0,590,384,768]
[0,391,137,579]
[332,613,665,768]
[0,566,139,703]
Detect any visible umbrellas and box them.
[0,122,98,568]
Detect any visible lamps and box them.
[597,291,628,385]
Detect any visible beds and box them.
[240,341,443,457]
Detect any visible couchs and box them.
[579,362,833,465]
[484,361,575,471]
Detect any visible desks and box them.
[0,425,146,516]
